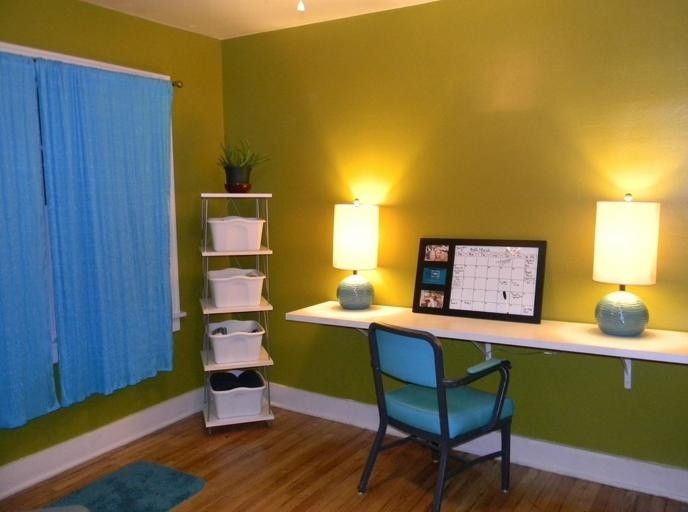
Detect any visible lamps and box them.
[332,199,380,310]
[593,193,660,338]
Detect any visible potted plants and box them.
[217,137,271,193]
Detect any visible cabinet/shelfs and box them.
[199,193,275,435]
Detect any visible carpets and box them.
[34,461,205,511]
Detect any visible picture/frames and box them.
[412,238,546,325]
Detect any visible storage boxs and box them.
[208,369,267,420]
[206,320,266,364]
[207,268,267,308]
[207,215,266,251]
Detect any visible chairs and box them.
[357,322,514,512]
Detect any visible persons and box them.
[421,292,443,307]
[424,247,449,262]
